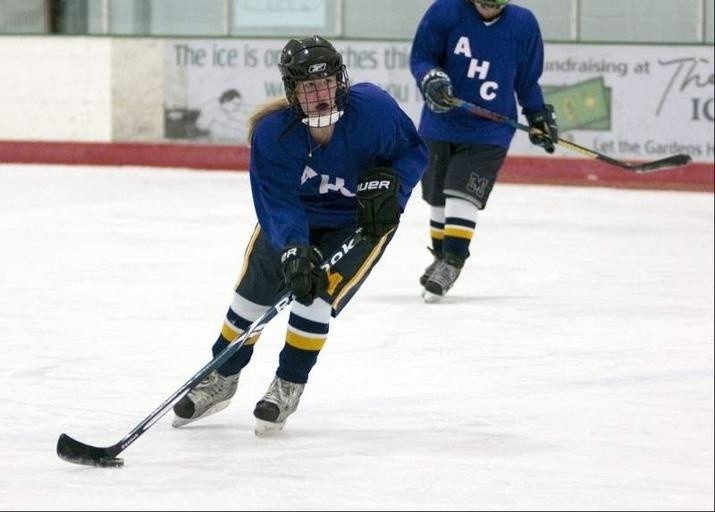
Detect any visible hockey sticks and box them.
[58,226,365,468]
[453,96,693,173]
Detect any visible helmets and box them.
[278,35,348,128]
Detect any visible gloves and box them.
[280,246,329,305]
[522,105,557,152]
[356,166,400,237]
[420,68,458,114]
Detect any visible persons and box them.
[408,1,560,296]
[173,32,432,425]
[191,85,250,143]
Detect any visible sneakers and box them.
[174,370,240,418]
[420,245,469,296]
[254,374,305,422]
